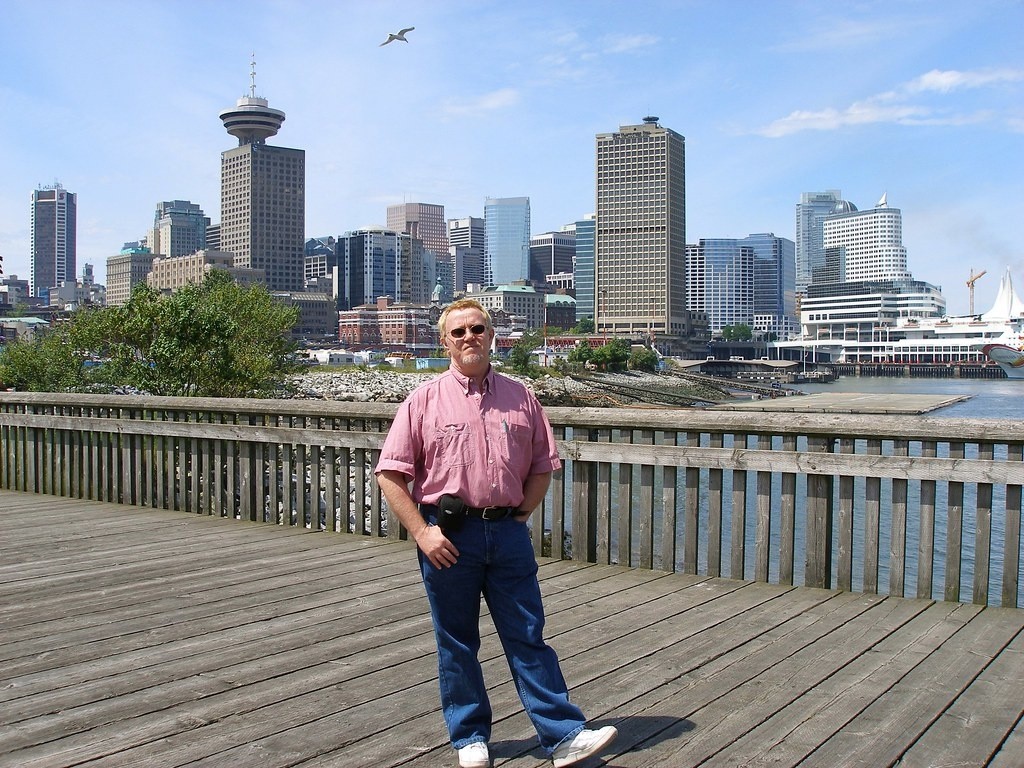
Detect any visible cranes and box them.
[966,269,987,314]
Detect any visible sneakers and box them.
[459,742,490,768]
[552,726,618,768]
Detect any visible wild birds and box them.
[379,26,415,47]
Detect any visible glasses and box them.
[446,324,486,338]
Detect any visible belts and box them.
[463,506,529,520]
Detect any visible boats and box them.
[970,312,1024,381]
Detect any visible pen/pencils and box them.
[502,419,508,432]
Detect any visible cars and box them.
[489,360,504,367]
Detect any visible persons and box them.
[374,298,620,768]
